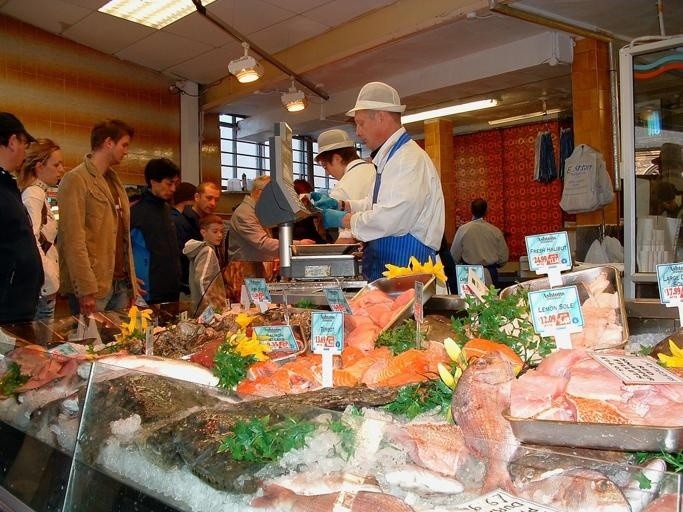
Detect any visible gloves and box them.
[312,192,338,210]
[323,209,348,228]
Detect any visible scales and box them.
[254,176,361,282]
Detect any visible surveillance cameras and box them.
[168,78,182,94]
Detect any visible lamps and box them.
[401,98,499,127]
[280,75,307,114]
[226,44,265,84]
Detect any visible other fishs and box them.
[252,482,413,512]
[622,458,666,511]
[351,462,463,493]
[384,421,487,489]
[451,348,521,497]
[514,468,630,510]
[507,442,644,491]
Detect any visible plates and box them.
[505,418,683,452]
[287,243,362,258]
[269,324,307,369]
[348,272,436,342]
[500,265,630,350]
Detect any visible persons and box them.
[0,112,45,325]
[170,182,197,221]
[272,180,340,244]
[228,176,315,303]
[450,198,509,290]
[651,143,683,194]
[655,182,683,264]
[14,138,65,320]
[57,119,147,315]
[314,129,377,244]
[129,159,182,305]
[182,215,226,315]
[309,81,448,296]
[177,182,224,302]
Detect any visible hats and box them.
[173,183,197,203]
[655,182,682,203]
[346,82,406,116]
[0,111,37,145]
[651,143,682,167]
[315,129,354,160]
